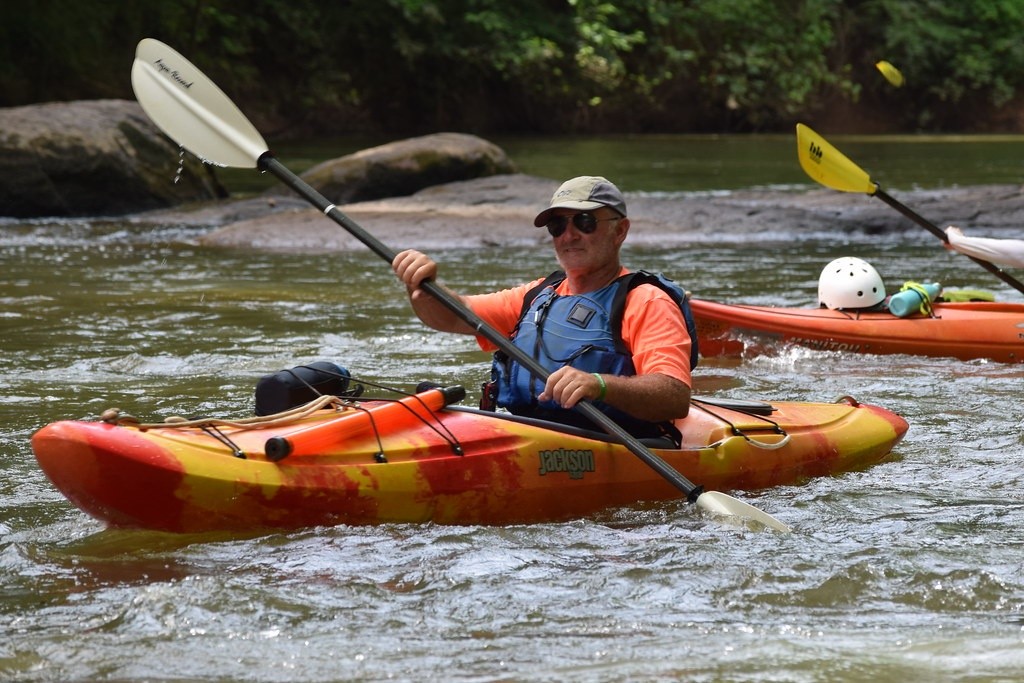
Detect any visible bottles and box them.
[889,282,943,317]
[256,362,351,415]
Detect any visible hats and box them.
[534,175,627,227]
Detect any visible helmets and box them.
[817,256,887,313]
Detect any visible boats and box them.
[685,294,1024,365]
[32,362,909,535]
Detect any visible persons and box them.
[392,176,698,448]
[942,226,1024,268]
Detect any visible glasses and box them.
[546,212,619,237]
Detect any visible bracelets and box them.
[591,372,608,407]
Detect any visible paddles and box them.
[130,36,796,535]
[796,124,1024,293]
